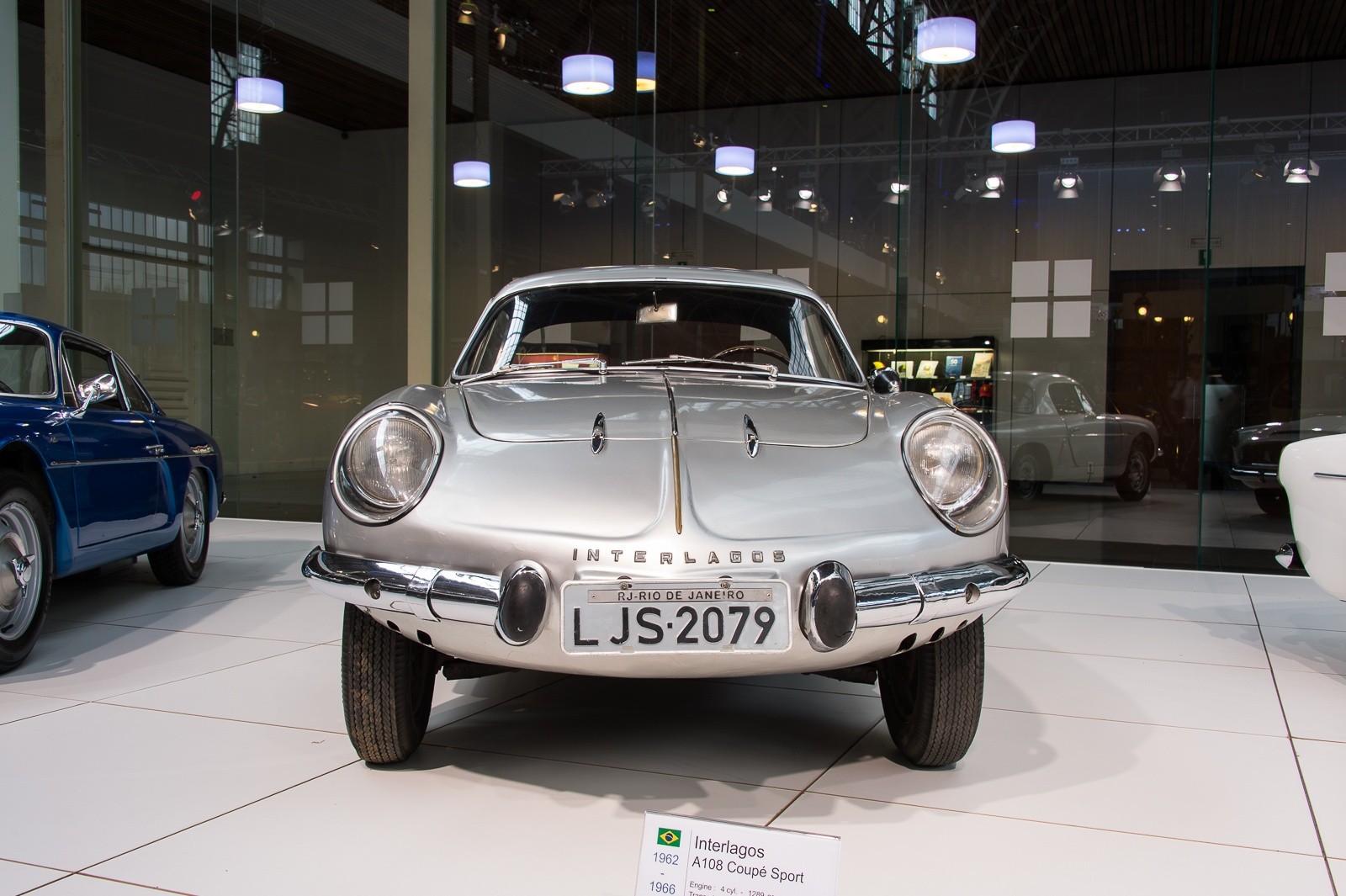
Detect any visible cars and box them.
[0,313,229,674]
[300,261,1033,769]
[951,370,1161,502]
[1225,413,1346,516]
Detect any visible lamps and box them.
[562,0,614,95]
[983,174,1001,190]
[236,0,283,114]
[1254,164,1271,179]
[597,177,615,205]
[991,25,1036,155]
[1153,161,1186,192]
[494,23,514,48]
[1053,171,1084,199]
[916,0,977,65]
[712,71,754,176]
[715,185,733,205]
[452,115,490,188]
[562,180,583,207]
[457,0,478,25]
[1284,157,1320,184]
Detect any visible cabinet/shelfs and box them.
[860,335,998,442]
